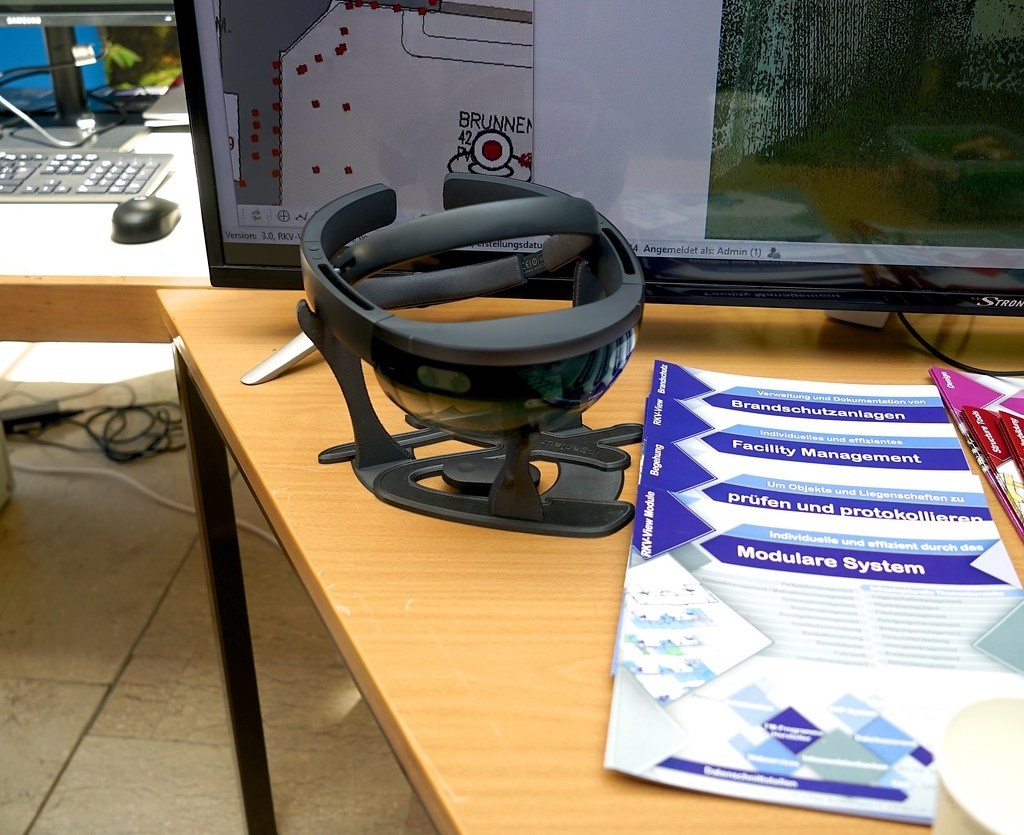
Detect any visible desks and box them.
[152,287,1024,835]
[0,85,210,344]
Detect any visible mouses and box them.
[111,195,181,243]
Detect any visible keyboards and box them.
[0,148,173,202]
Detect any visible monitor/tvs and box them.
[0,0,177,150]
[175,0,1024,326]
